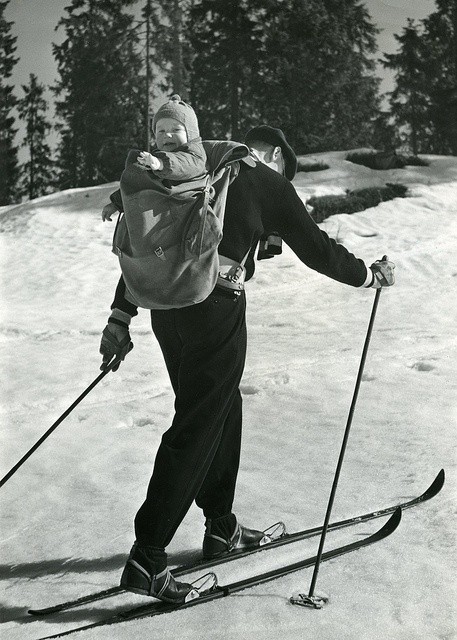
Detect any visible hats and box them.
[151,94,200,143]
[244,124,297,181]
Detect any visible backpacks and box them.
[112,140,250,310]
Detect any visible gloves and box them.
[99,323,131,371]
[368,260,396,289]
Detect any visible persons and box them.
[101,95,207,222]
[100,124,396,605]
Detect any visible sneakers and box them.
[203,528,266,559]
[120,558,193,604]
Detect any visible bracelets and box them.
[365,270,375,288]
